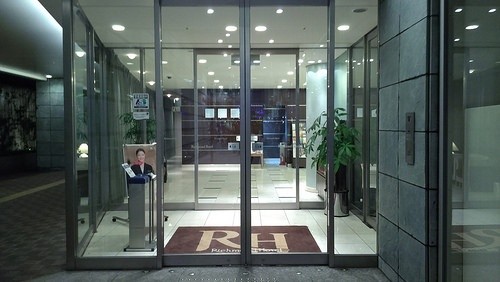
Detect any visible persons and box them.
[127,147,154,189]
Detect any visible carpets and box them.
[164,226,321,254]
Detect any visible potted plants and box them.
[303,108,361,217]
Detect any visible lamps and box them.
[77,143,88,157]
